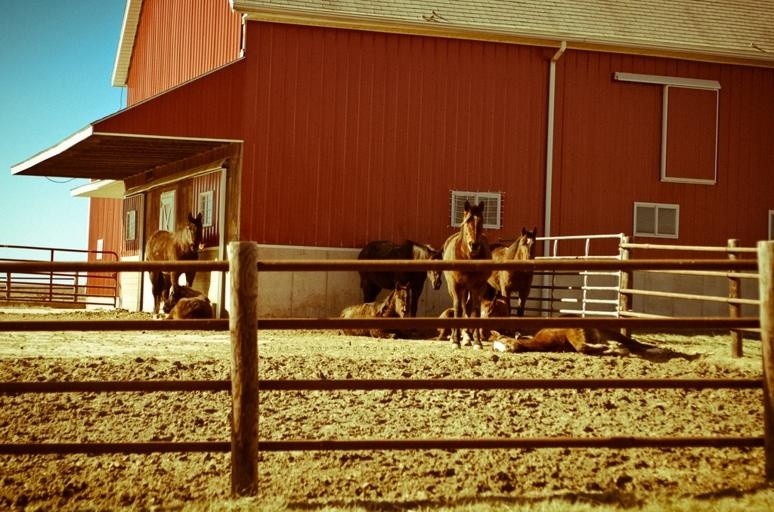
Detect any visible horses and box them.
[486,225,538,316]
[441,199,493,352]
[437,295,509,342]
[357,240,444,317]
[491,313,657,355]
[161,284,213,320]
[338,280,413,339]
[144,211,203,320]
